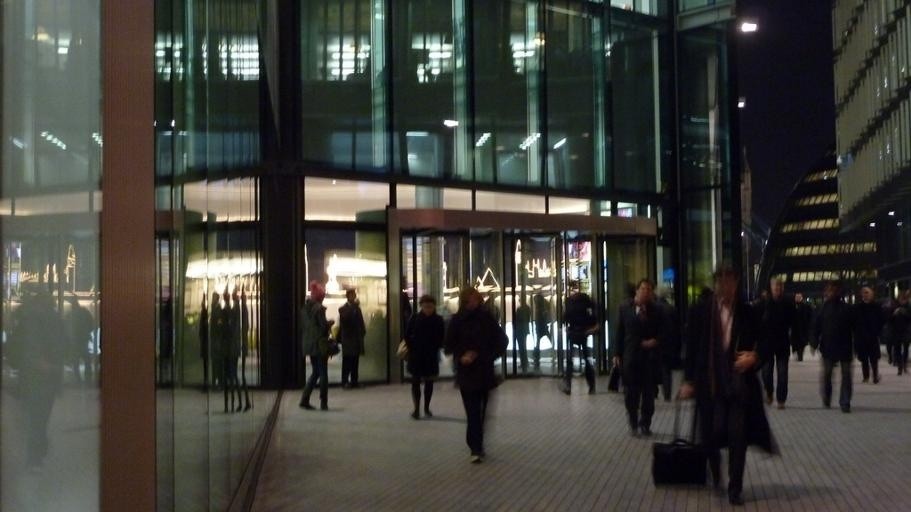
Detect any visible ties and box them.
[637,304,645,323]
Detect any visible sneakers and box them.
[728,492,746,506]
[630,423,654,437]
[777,401,786,409]
[712,479,726,499]
[299,402,316,412]
[471,449,486,464]
[320,401,328,410]
[766,394,774,405]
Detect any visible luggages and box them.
[650,389,710,487]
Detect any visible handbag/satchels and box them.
[396,338,409,362]
[608,362,621,393]
[327,321,340,357]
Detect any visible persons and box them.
[443,283,509,466]
[482,290,501,329]
[296,278,334,414]
[336,288,369,389]
[681,267,781,508]
[396,291,443,419]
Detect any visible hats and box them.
[310,279,326,300]
